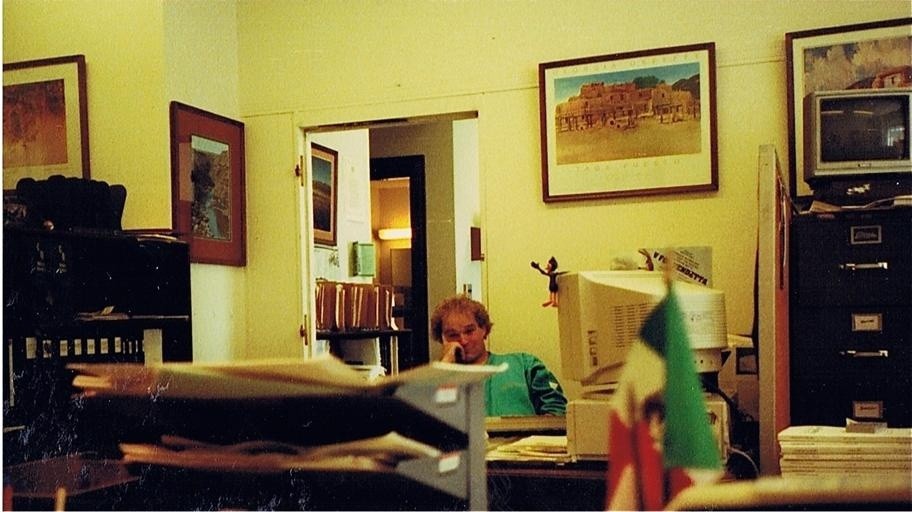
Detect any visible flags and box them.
[604,289,726,511]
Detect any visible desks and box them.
[485,434,736,512]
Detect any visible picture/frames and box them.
[538,42,720,203]
[3,54,91,190]
[784,16,912,205]
[312,141,339,247]
[169,100,247,267]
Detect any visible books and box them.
[497,433,567,453]
[776,424,912,479]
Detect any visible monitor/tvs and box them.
[809,88,912,176]
[558,269,727,388]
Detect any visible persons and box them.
[530,256,570,307]
[430,295,568,418]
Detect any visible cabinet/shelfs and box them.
[316,328,412,377]
[3,175,192,431]
[66,365,487,512]
[789,209,912,427]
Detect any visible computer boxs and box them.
[565,393,733,465]
